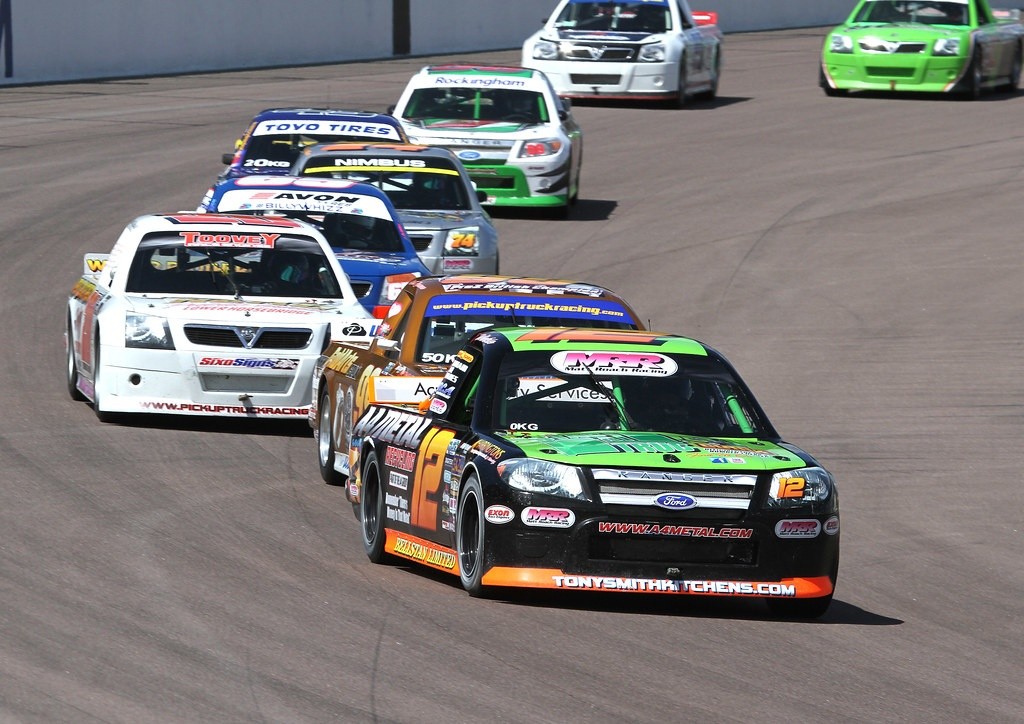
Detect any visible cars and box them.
[819,0,1024,101]
[387,64,584,218]
[65,107,501,418]
[520,0,726,110]
[308,276,842,618]
